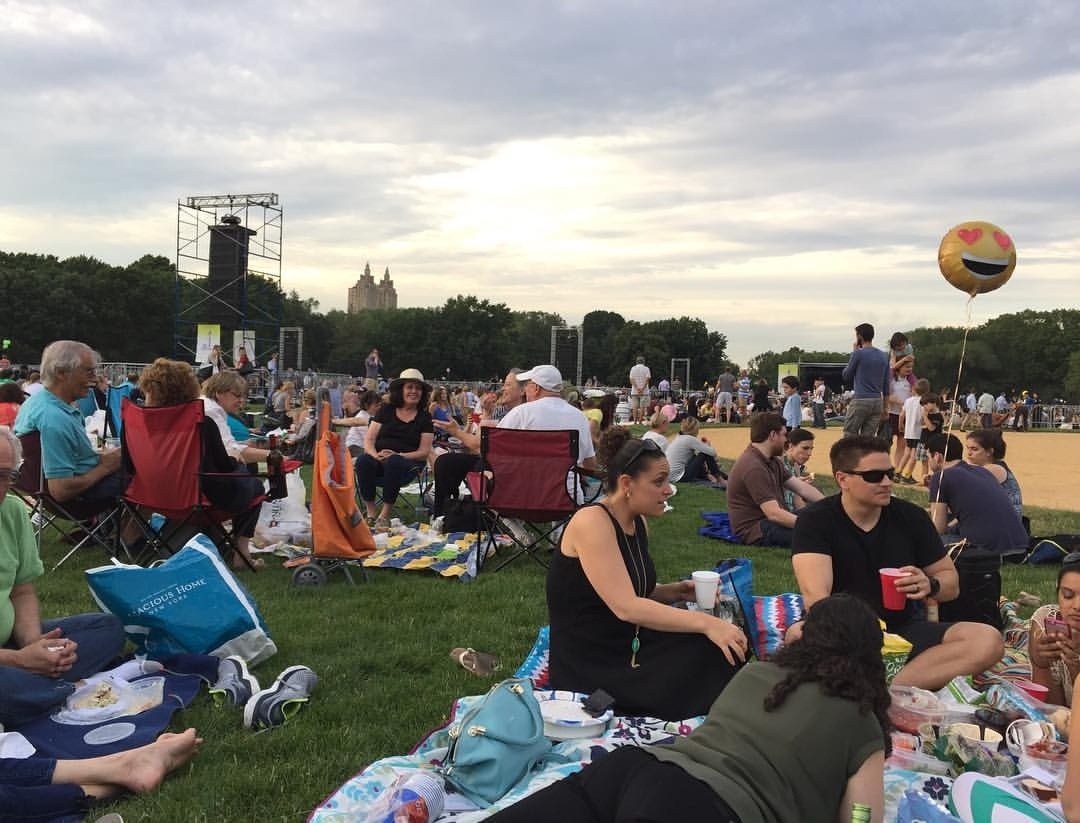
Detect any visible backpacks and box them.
[820,384,832,402]
[932,541,1002,631]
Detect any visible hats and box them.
[516,365,563,393]
[389,368,433,394]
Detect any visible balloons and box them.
[938,222,1017,296]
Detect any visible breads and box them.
[1020,779,1057,801]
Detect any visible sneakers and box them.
[899,474,919,485]
[893,472,902,483]
[208,655,260,706]
[244,665,315,728]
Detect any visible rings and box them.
[736,626,743,632]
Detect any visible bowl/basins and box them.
[950,720,1003,758]
[120,674,164,715]
[885,684,945,734]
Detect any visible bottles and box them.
[266,435,288,500]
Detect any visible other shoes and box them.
[105,535,159,561]
[375,519,392,534]
[231,557,265,572]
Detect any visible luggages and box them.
[290,387,377,588]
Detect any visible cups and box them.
[104,436,120,449]
[94,813,127,823]
[1015,680,1048,701]
[390,518,401,527]
[692,570,721,610]
[879,567,909,610]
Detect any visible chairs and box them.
[10,384,306,577]
[353,425,605,577]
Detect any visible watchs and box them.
[925,573,941,599]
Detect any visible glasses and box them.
[74,367,97,378]
[1,471,19,485]
[621,438,662,474]
[841,466,895,483]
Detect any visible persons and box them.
[546,426,749,722]
[1,322,1080,823]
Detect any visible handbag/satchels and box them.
[678,557,759,661]
[435,677,580,807]
[85,532,278,675]
[256,468,307,549]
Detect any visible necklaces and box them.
[608,498,647,668]
